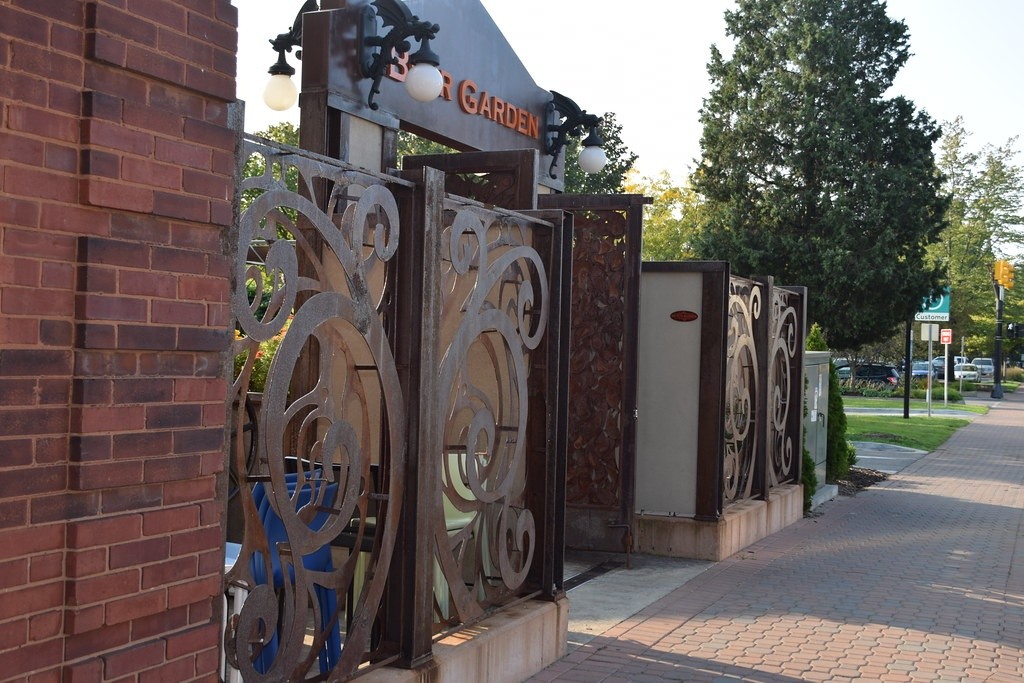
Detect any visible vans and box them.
[933,355,970,379]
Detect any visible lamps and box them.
[264,0,319,111]
[546,89,607,179]
[368,0,444,110]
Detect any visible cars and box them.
[911,360,938,381]
[970,357,995,375]
[832,358,862,368]
[953,364,981,383]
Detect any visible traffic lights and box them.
[1004,263,1014,289]
[999,260,1008,286]
[1006,322,1015,338]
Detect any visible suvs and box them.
[834,363,902,387]
